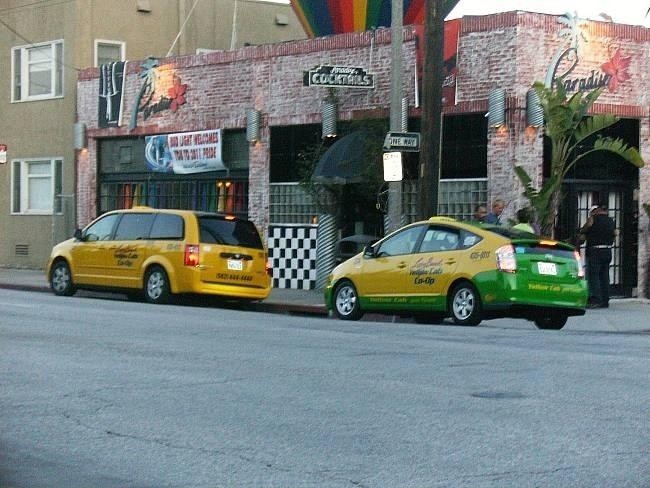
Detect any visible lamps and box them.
[246,106,259,143]
[401,97,408,134]
[73,121,90,151]
[488,87,505,127]
[321,100,336,139]
[527,86,547,128]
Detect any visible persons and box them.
[472,197,541,237]
[575,204,616,309]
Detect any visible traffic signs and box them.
[384,133,418,151]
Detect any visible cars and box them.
[326,213,589,327]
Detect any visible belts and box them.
[591,244,613,249]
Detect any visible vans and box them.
[47,207,277,304]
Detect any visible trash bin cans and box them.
[334,234,380,266]
